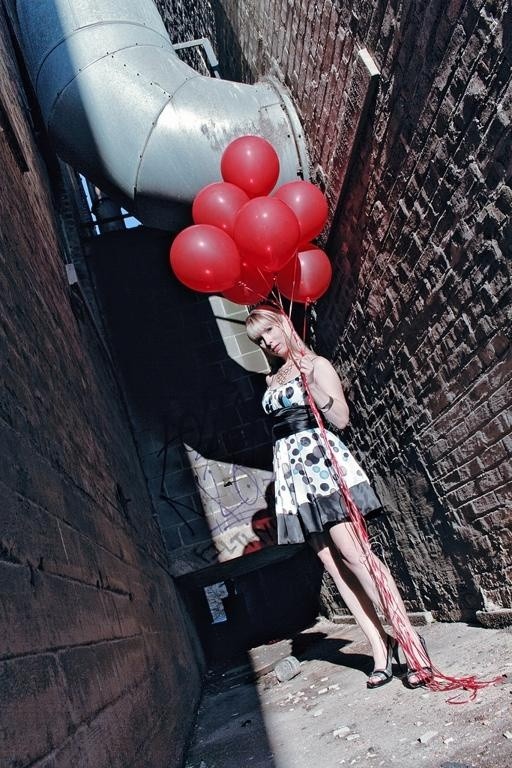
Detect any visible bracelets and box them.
[320,395,334,413]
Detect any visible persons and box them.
[241,303,437,690]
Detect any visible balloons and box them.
[169,136,336,306]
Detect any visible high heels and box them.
[365,633,402,690]
[402,630,432,689]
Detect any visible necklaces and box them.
[276,361,294,385]
